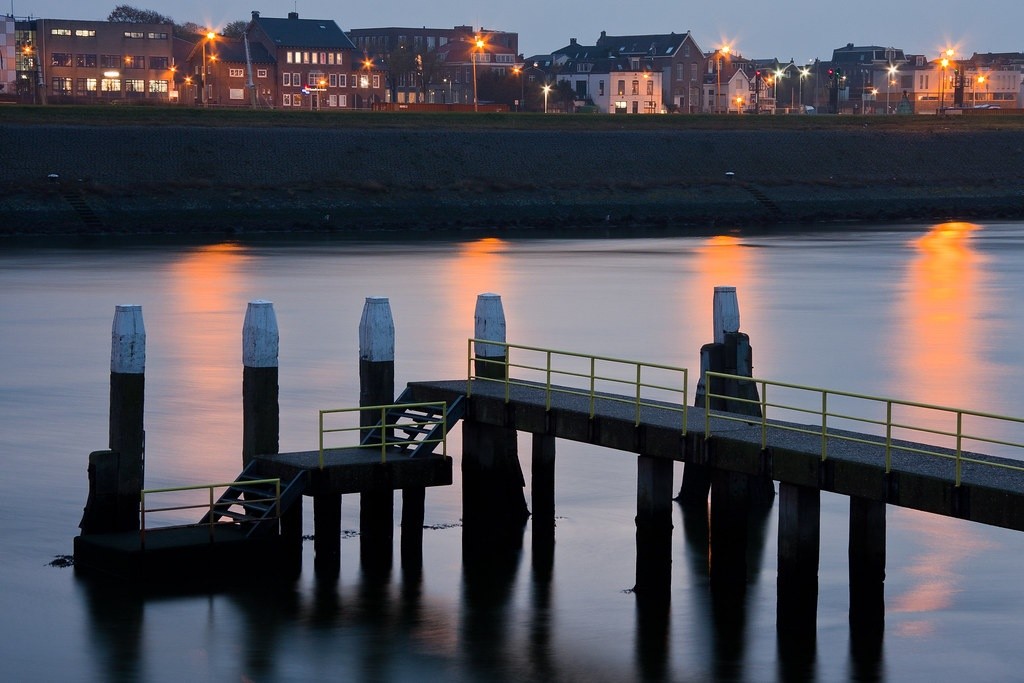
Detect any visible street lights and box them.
[316,79,327,111]
[202,31,220,108]
[938,49,955,109]
[360,56,375,111]
[716,45,730,113]
[885,63,899,113]
[168,65,177,104]
[23,45,33,104]
[543,82,550,114]
[184,76,192,106]
[644,74,652,113]
[765,70,808,108]
[870,88,878,113]
[736,97,742,114]
[973,71,986,107]
[514,68,524,112]
[124,56,133,104]
[472,38,484,112]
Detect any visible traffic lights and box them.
[757,69,762,81]
[829,67,840,81]
